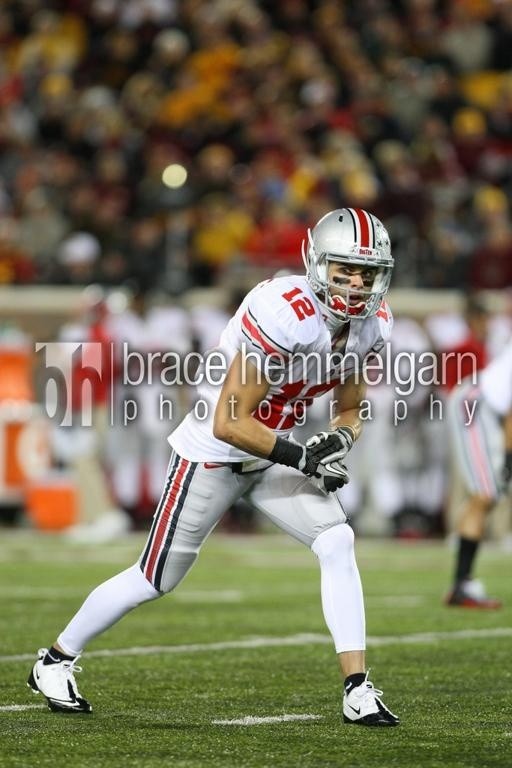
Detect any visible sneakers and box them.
[26,646,92,714]
[446,586,502,611]
[342,681,400,727]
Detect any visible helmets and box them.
[301,207,396,321]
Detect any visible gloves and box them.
[306,423,356,463]
[268,436,349,497]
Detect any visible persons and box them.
[447,341,510,611]
[0,0,511,536]
[26,205,400,725]
